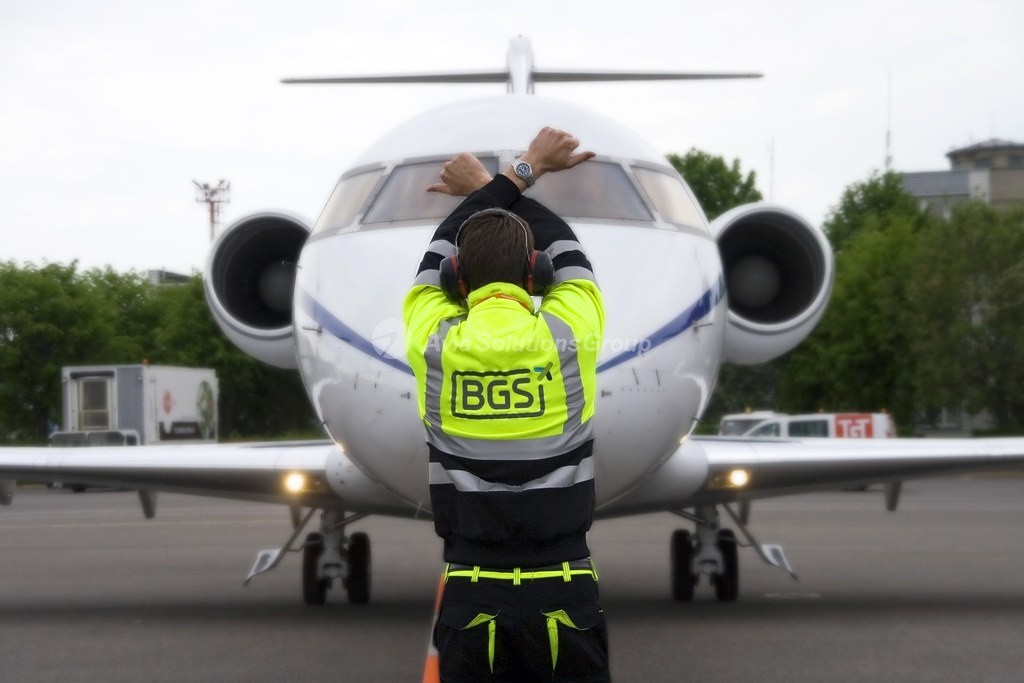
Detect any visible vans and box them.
[716,404,900,455]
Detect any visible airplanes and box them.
[0,28,1024,607]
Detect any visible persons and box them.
[402,123,612,683]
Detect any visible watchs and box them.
[508,159,537,188]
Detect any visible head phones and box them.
[437,207,555,301]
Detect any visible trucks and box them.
[42,359,223,493]
